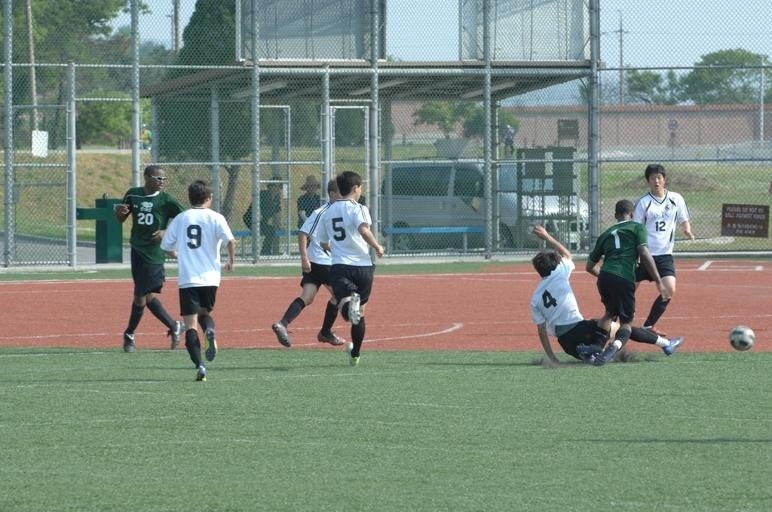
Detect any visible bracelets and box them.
[112,204,116,212]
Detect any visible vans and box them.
[374,157,597,256]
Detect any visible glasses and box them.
[151,176,167,181]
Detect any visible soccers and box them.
[730,326,755,351]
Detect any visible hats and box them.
[300,175,321,189]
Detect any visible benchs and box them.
[231,227,487,255]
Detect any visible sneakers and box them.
[641,325,666,337]
[197,368,206,381]
[124,330,135,352]
[272,322,291,347]
[204,327,217,361]
[348,292,361,325]
[575,343,602,354]
[318,330,346,345]
[346,341,360,366]
[168,320,186,349]
[662,336,683,355]
[594,342,618,366]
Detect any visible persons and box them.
[160,179,235,382]
[530,225,684,364]
[242,176,283,255]
[272,180,346,347]
[296,175,321,248]
[114,165,186,354]
[139,124,151,149]
[504,125,514,156]
[632,164,695,337]
[317,171,384,366]
[583,200,671,366]
